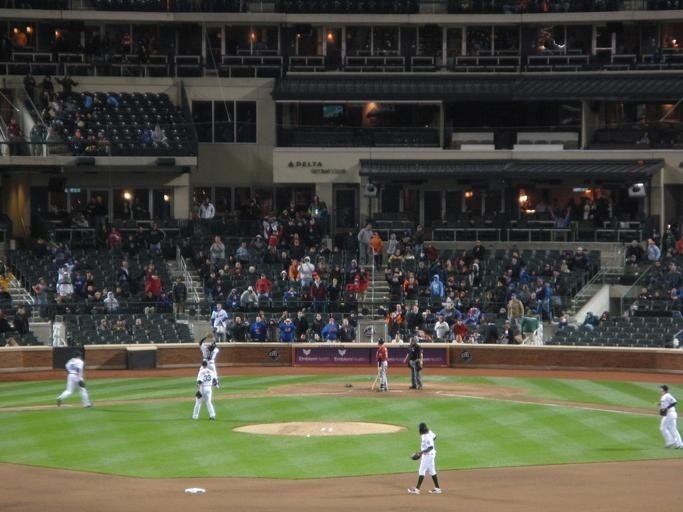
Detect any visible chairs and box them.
[41,90,190,156]
[7,237,357,344]
[387,248,683,347]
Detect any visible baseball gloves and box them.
[195,391,202,400]
[411,452,421,460]
[658,408,666,416]
[206,332,213,340]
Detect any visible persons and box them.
[57,350,93,408]
[659,384,683,449]
[407,423,442,495]
[192,332,219,420]
[376,336,423,391]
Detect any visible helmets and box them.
[420,424,427,433]
[660,384,670,393]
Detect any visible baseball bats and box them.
[371,367,383,391]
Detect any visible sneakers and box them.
[408,488,420,494]
[428,488,442,494]
